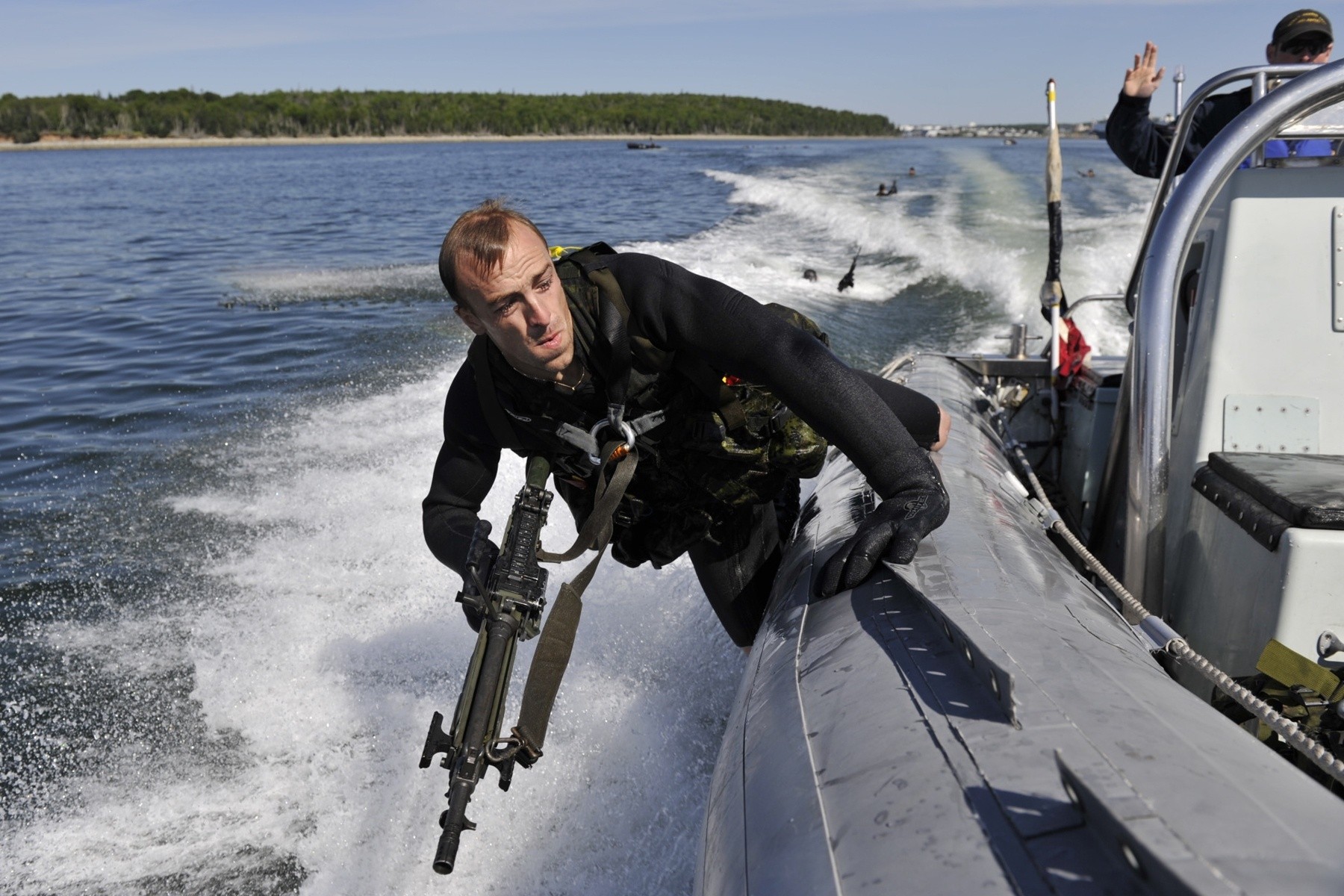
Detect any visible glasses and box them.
[1288,38,1328,54]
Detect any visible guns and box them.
[418,456,554,877]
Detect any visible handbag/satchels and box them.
[711,302,830,479]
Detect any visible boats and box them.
[628,142,661,149]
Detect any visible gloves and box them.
[814,452,949,598]
[457,520,505,631]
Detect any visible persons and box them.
[422,196,952,657]
[1104,9,1333,178]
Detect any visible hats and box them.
[1272,9,1334,47]
